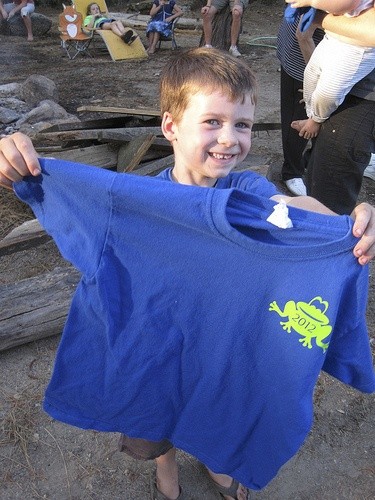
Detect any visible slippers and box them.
[205,469,249,500]
[152,468,185,500]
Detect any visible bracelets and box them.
[206,5,210,8]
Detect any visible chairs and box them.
[58,4,101,61]
[71,0,150,63]
[146,12,180,51]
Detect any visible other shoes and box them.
[121,30,133,43]
[285,177,308,197]
[230,46,242,58]
[127,36,138,45]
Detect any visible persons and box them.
[84,3,139,44]
[0,49,375,500]
[0,0,35,41]
[202,0,245,57]
[285,0,375,139]
[146,0,183,53]
[275,6,324,196]
[305,8,375,215]
